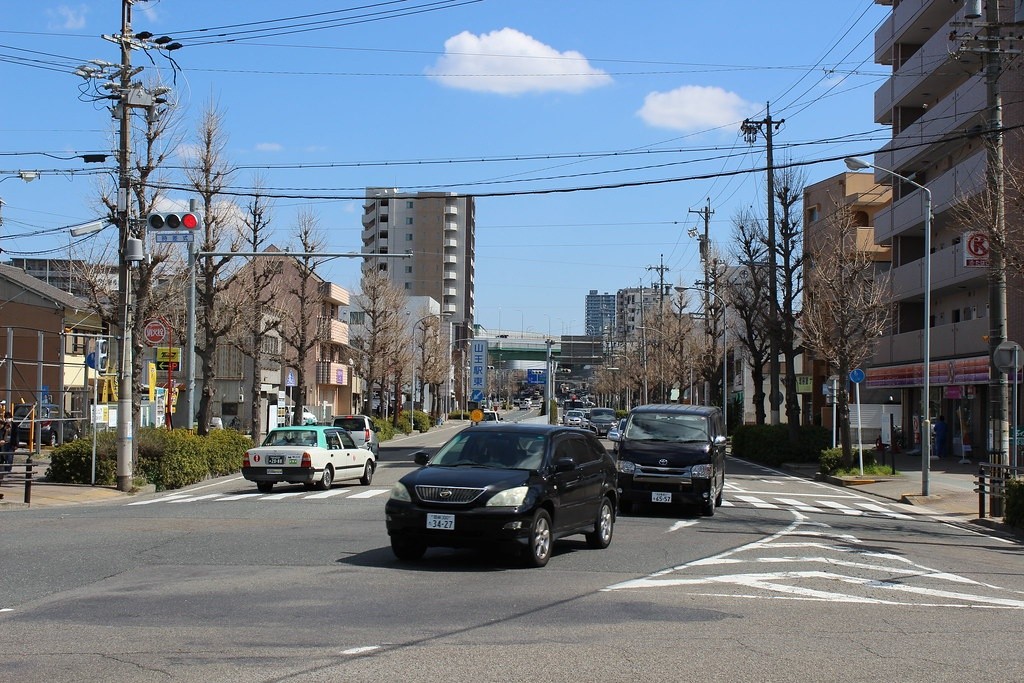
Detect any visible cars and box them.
[238,426,377,490]
[512,386,628,450]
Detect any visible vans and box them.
[332,415,380,460]
[612,405,728,517]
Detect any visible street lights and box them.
[611,355,631,412]
[411,312,453,432]
[675,286,728,442]
[636,325,693,406]
[445,337,474,421]
[845,156,932,497]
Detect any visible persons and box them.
[934,415,949,457]
[0,412,19,500]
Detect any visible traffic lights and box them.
[147,211,203,230]
[531,370,543,374]
[95,339,108,373]
[557,368,571,373]
[485,366,494,370]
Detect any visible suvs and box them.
[384,424,619,567]
[469,410,504,426]
[13,403,82,447]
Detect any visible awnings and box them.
[301,412,317,426]
